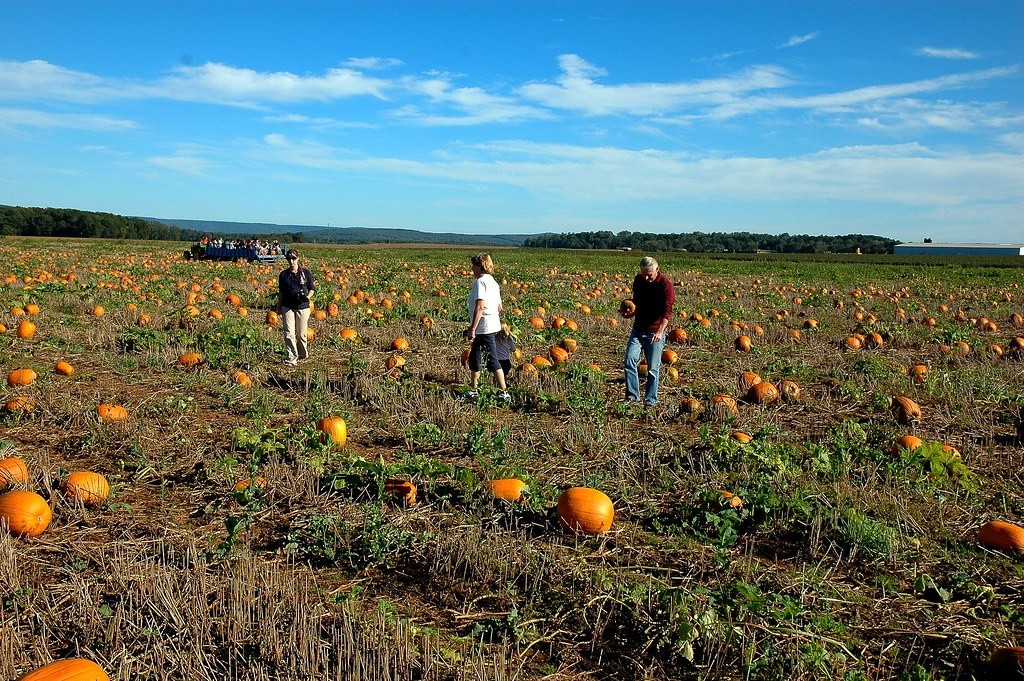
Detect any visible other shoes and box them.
[498,391,511,399]
[644,403,653,410]
[463,389,479,397]
[622,398,639,407]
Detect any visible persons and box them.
[466,253,516,401]
[621,256,674,410]
[201,233,282,255]
[278,249,317,368]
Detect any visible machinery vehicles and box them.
[183,231,287,265]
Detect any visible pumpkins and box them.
[14,659,112,681]
[0,253,1024,557]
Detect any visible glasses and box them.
[286,256,298,261]
